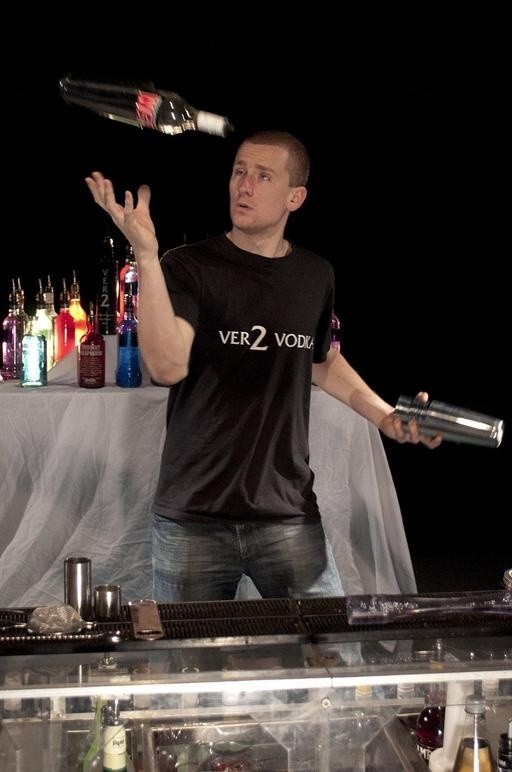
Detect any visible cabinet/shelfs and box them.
[0,636,512,772]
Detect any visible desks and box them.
[0,335,420,608]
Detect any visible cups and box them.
[394,397,504,450]
[63,557,122,621]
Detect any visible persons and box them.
[85,123,448,600]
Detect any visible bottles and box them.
[59,76,234,139]
[2,238,142,388]
[103,700,128,772]
[410,694,512,772]
